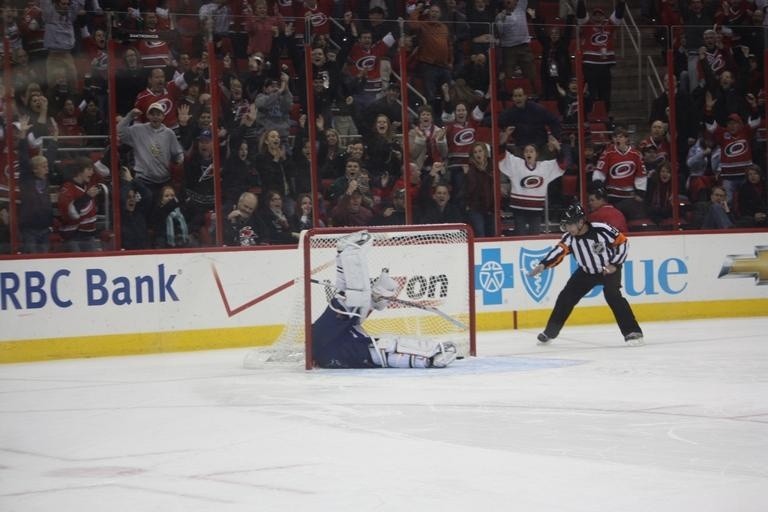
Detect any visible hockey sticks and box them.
[310,279,467,331]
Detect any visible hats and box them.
[593,9,604,15]
[147,103,165,114]
[264,78,279,85]
[388,82,401,90]
[251,52,264,62]
[728,113,741,121]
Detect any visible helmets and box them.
[561,204,585,223]
[434,341,463,359]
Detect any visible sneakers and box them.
[538,332,551,343]
[430,351,444,360]
[337,231,370,252]
[625,332,644,341]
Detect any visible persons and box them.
[320,129,350,180]
[498,126,567,236]
[704,91,761,201]
[551,77,595,148]
[413,162,462,223]
[218,79,253,141]
[527,5,576,84]
[347,96,403,191]
[441,83,490,166]
[254,71,294,146]
[152,186,200,249]
[637,162,675,223]
[567,134,599,197]
[302,0,332,45]
[493,0,543,100]
[682,0,713,48]
[89,27,108,66]
[273,0,301,57]
[129,10,178,72]
[135,62,206,141]
[242,0,280,62]
[698,46,749,125]
[18,115,58,252]
[343,12,400,92]
[75,0,104,37]
[364,7,385,29]
[408,3,451,99]
[451,79,481,106]
[23,82,41,107]
[176,104,212,138]
[23,2,43,27]
[733,163,768,227]
[697,29,730,89]
[741,8,766,52]
[225,140,254,191]
[685,130,721,201]
[0,5,38,64]
[443,34,505,122]
[689,186,736,230]
[223,51,291,100]
[1,120,40,224]
[744,88,767,153]
[526,202,645,345]
[331,181,397,227]
[368,83,405,136]
[297,47,353,128]
[59,156,100,250]
[190,0,230,59]
[290,193,324,239]
[591,127,647,220]
[259,192,295,245]
[81,100,110,146]
[574,0,626,121]
[468,0,497,36]
[290,114,326,194]
[496,86,564,161]
[408,104,449,174]
[640,120,670,174]
[457,142,508,238]
[586,188,628,233]
[94,149,112,176]
[323,158,375,208]
[308,227,458,369]
[120,165,155,251]
[388,164,421,211]
[182,130,226,215]
[115,47,152,116]
[255,127,298,196]
[13,49,41,99]
[39,0,81,82]
[651,72,693,136]
[345,140,364,161]
[49,76,73,110]
[201,191,257,246]
[116,102,185,189]
